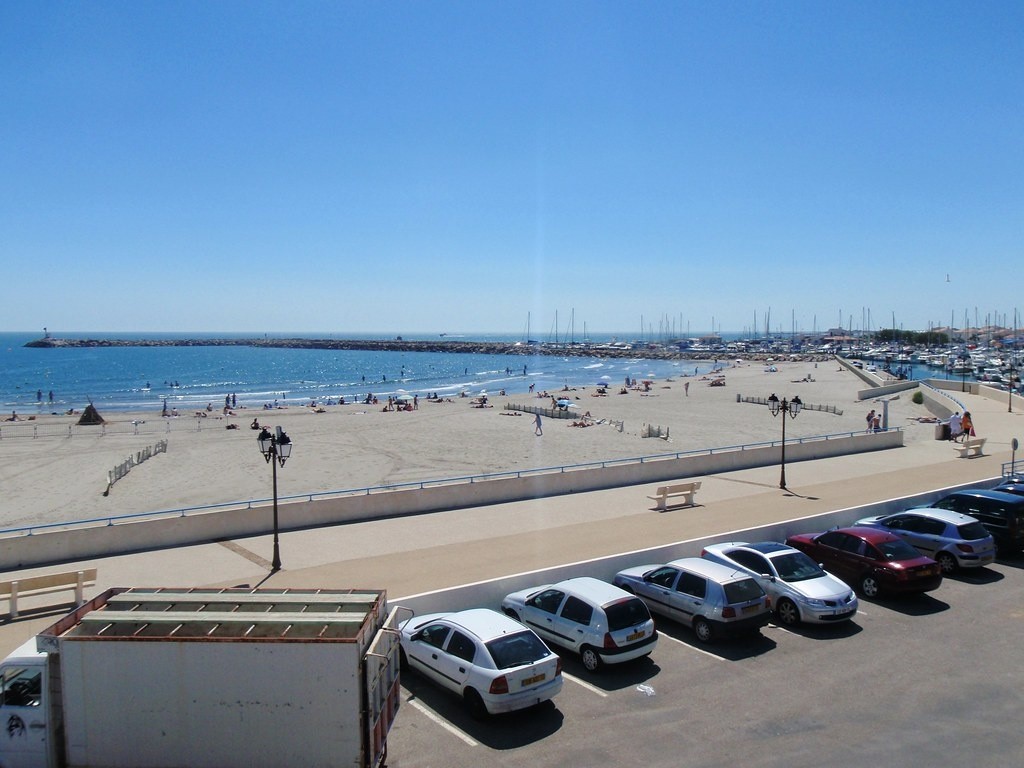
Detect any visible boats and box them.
[526,306,1024,398]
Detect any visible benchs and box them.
[953,438,988,457]
[648,481,702,510]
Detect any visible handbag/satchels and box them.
[970,428,975,436]
[967,421,971,426]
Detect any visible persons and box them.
[866,410,882,433]
[561,365,737,398]
[949,412,973,444]
[6,364,559,431]
[531,414,544,435]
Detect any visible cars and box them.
[856,507,997,588]
[611,555,775,645]
[789,526,943,601]
[698,540,858,629]
[904,488,1024,559]
[995,474,1024,495]
[397,607,564,721]
[501,574,661,673]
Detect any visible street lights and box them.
[257,425,292,572]
[1005,361,1015,412]
[766,392,803,489]
[957,354,971,392]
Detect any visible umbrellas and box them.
[395,389,408,396]
[600,375,611,381]
[597,381,608,387]
[478,393,487,403]
[397,395,413,404]
[559,399,573,410]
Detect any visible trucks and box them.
[1,586,415,768]
